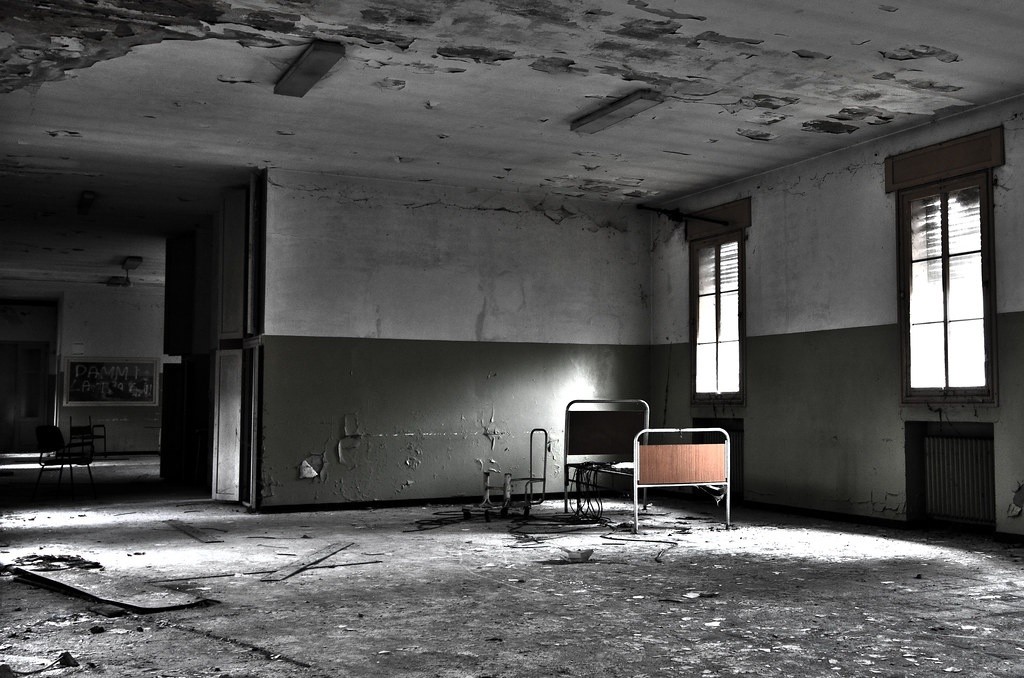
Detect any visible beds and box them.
[564,399,731,533]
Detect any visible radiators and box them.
[923,437,995,526]
[703,429,743,494]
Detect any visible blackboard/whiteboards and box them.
[61,357,160,408]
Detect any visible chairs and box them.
[59,425,95,488]
[35,424,71,490]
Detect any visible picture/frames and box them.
[61,356,160,407]
